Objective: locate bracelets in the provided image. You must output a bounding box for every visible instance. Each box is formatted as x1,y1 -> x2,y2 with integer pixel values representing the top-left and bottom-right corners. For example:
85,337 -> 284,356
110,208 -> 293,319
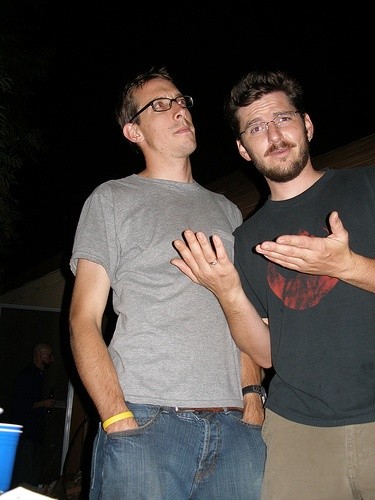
103,411 -> 134,430
242,385 -> 267,403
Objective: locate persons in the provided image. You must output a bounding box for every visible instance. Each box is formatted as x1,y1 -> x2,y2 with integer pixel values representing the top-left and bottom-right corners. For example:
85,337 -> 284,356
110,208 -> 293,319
68,67 -> 268,500
169,72 -> 375,500
12,343 -> 56,479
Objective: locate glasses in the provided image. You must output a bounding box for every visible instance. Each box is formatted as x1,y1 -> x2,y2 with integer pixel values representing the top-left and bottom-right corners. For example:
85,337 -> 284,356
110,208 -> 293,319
237,110 -> 300,137
124,95 -> 193,121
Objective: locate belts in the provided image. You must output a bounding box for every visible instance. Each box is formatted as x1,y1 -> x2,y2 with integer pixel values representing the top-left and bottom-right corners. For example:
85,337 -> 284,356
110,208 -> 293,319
163,401 -> 246,415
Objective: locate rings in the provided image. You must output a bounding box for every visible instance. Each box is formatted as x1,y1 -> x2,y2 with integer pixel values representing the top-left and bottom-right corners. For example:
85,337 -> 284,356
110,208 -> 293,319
209,260 -> 217,265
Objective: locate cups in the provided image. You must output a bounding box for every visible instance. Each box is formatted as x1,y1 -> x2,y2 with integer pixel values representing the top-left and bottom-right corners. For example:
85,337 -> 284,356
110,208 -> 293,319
0,423 -> 23,492
